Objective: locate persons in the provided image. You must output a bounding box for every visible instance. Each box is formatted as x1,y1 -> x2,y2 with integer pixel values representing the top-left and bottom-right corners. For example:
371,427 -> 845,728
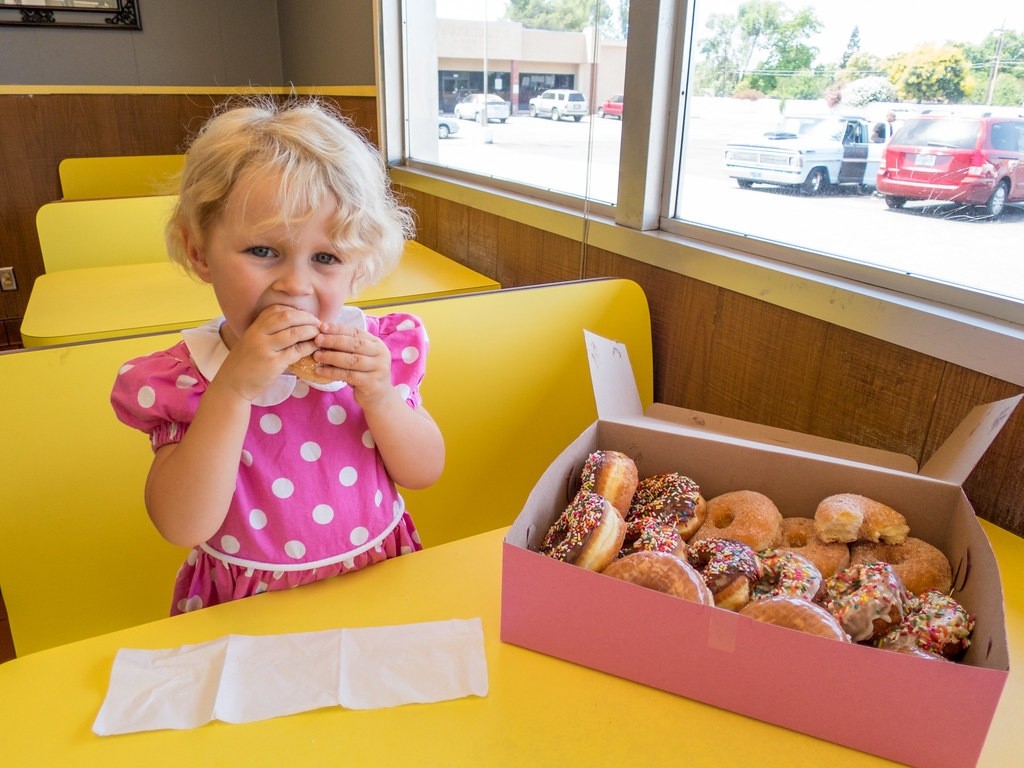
870,112 -> 896,143
109,108 -> 445,617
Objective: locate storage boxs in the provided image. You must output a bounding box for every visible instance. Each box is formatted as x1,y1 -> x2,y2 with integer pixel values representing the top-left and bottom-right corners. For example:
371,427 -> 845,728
500,328 -> 1023,768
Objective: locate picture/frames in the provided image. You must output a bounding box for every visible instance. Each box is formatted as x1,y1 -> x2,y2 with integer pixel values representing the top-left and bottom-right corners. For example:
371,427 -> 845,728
0,0 -> 143,32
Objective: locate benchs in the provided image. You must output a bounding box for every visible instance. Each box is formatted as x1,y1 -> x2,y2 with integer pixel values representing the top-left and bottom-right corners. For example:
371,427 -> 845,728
36,195 -> 183,272
0,277 -> 656,657
59,155 -> 188,198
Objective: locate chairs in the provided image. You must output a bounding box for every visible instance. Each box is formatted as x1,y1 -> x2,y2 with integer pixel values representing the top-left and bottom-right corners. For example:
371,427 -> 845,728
845,125 -> 856,142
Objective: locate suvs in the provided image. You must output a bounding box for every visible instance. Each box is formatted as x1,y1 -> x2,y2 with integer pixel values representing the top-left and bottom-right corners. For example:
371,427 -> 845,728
529,89 -> 588,123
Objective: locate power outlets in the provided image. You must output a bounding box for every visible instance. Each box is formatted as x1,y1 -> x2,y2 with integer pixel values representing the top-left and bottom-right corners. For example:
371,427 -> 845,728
0,267 -> 18,290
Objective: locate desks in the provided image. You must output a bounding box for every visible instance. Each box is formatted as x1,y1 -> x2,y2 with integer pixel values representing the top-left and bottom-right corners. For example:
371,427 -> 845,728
0,516 -> 1023,768
21,239 -> 501,348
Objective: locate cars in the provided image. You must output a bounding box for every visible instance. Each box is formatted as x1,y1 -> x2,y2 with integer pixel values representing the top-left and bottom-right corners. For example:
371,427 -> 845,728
454,93 -> 513,124
439,114 -> 460,139
874,113 -> 1024,221
597,94 -> 624,121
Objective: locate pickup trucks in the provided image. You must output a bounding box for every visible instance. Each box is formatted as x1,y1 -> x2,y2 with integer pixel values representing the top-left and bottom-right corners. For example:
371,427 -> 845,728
724,113 -> 894,197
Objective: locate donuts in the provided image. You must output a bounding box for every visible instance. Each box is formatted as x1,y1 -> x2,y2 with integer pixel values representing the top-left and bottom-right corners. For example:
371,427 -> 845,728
291,348 -> 340,385
542,449 -> 977,662
